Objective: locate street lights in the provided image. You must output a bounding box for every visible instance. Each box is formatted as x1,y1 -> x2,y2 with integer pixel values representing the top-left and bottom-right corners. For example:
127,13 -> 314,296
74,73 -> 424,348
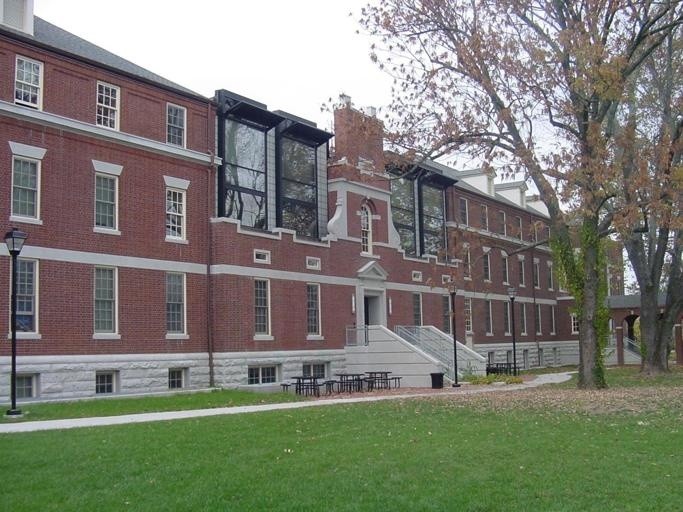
5,226 -> 30,415
507,284 -> 518,376
448,282 -> 461,388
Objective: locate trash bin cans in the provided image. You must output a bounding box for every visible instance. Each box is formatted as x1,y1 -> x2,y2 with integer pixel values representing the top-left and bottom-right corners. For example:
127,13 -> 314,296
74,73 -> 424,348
430,373 -> 444,389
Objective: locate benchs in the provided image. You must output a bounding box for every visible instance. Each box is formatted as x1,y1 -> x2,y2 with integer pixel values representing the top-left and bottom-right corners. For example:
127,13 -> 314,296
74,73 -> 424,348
486,362 -> 522,376
280,372 -> 403,398
